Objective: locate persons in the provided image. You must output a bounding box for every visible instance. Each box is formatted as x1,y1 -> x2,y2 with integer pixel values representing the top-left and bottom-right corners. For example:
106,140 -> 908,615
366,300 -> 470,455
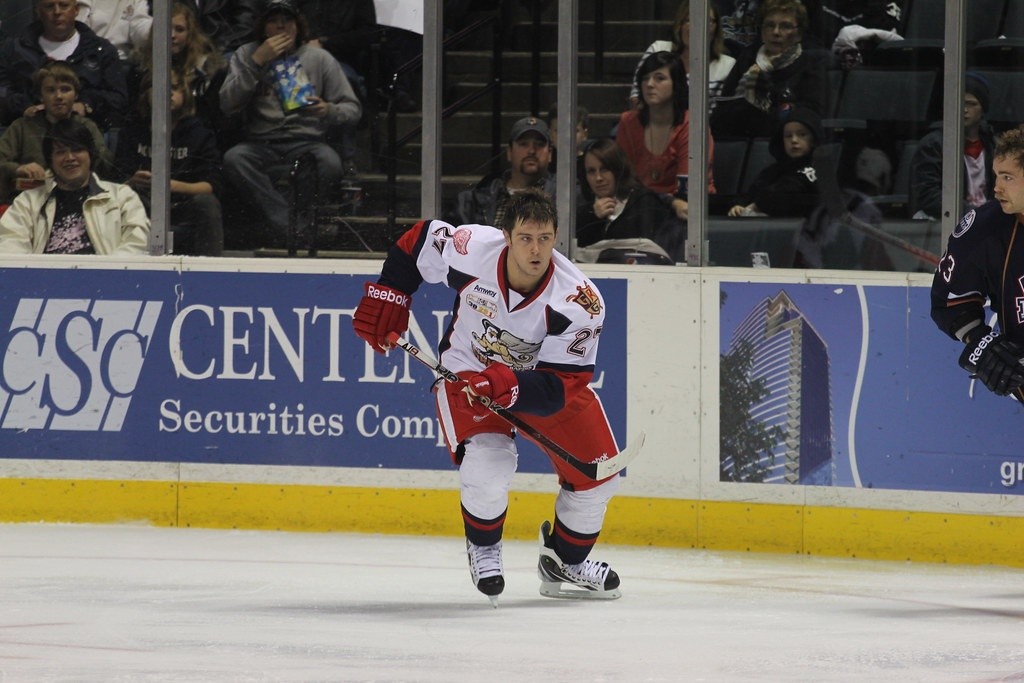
908,75 -> 998,217
294,0 -> 376,176
443,118 -> 687,230
630,0 -> 737,119
114,68 -> 224,257
801,0 -> 901,68
793,145 -> 897,271
727,108 -> 837,218
220,0 -> 363,248
353,189 -> 621,607
577,139 -> 682,265
75,0 -> 154,62
0,62 -> 115,205
614,51 -> 716,199
0,0 -> 128,138
929,124 -> 1024,407
713,0 -> 840,138
0,120 -> 151,255
124,0 -> 226,124
547,102 -> 594,182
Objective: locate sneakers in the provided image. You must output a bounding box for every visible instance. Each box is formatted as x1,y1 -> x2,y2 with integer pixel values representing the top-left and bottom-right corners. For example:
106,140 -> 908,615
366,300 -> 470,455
465,533 -> 505,609
536,521 -> 620,600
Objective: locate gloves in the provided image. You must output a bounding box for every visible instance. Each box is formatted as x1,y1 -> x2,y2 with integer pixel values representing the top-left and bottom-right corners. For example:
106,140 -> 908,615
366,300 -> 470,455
451,363 -> 519,418
351,281 -> 412,354
958,326 -> 1024,395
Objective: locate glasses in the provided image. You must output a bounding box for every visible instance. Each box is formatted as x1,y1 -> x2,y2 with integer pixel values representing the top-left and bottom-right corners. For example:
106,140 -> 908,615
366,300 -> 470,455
761,22 -> 801,33
683,17 -> 717,25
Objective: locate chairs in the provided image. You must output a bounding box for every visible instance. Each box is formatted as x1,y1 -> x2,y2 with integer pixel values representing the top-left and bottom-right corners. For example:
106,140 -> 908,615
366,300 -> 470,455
169,1 -> 1024,251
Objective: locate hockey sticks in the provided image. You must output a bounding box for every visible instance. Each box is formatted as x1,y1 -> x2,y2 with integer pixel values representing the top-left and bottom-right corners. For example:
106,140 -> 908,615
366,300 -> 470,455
386,331 -> 645,481
812,140 -> 942,264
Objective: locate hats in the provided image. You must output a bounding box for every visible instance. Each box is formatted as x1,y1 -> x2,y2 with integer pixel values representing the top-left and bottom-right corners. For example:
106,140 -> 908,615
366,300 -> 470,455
956,78 -> 989,113
511,116 -> 550,141
261,0 -> 298,18
